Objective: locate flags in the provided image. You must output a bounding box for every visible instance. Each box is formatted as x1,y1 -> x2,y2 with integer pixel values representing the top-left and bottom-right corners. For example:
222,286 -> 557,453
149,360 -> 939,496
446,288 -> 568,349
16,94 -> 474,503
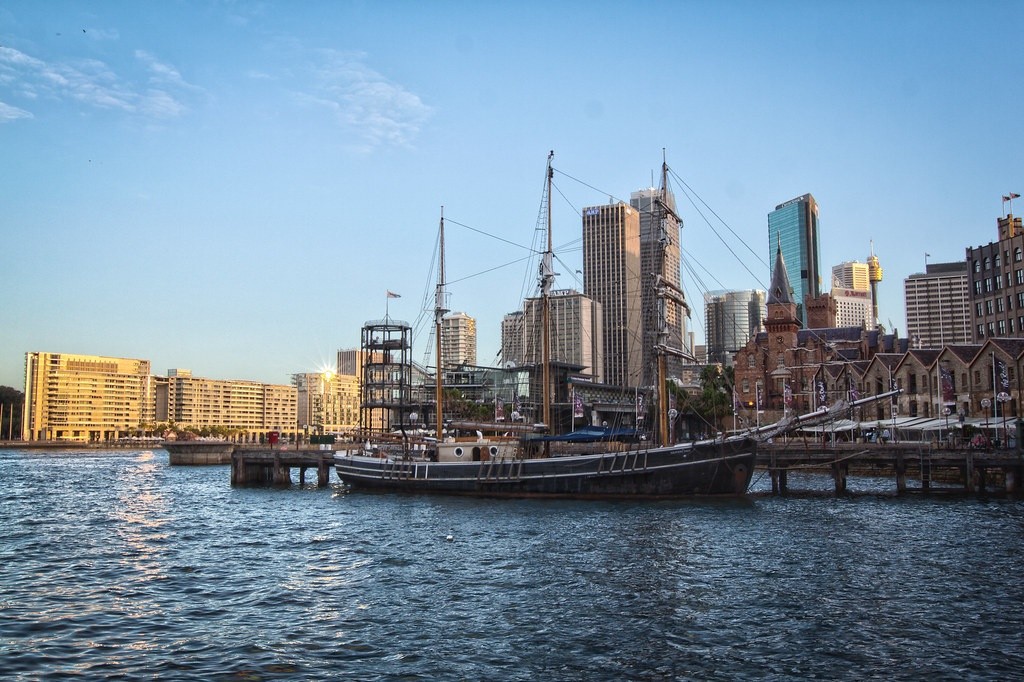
1003,196 -> 1010,201
851,379 -> 856,400
734,394 -> 738,410
576,270 -> 582,274
513,393 -> 522,412
784,385 -> 792,407
926,254 -> 929,256
891,377 -> 897,405
940,365 -> 954,400
1011,193 -> 1020,198
637,395 -> 644,419
574,392 -> 583,418
815,381 -> 826,401
387,291 -> 400,298
497,395 -> 505,420
670,394 -> 676,409
994,357 -> 1008,392
758,386 -> 762,405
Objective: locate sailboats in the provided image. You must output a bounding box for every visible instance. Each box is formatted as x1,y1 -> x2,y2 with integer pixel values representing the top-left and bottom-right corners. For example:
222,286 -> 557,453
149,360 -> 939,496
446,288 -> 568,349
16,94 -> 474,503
330,144 -> 904,510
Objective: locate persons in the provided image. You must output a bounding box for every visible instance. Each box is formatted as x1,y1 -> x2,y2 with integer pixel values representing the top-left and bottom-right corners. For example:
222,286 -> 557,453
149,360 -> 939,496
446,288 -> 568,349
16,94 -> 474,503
1006,425 -> 1010,448
853,425 -> 889,443
